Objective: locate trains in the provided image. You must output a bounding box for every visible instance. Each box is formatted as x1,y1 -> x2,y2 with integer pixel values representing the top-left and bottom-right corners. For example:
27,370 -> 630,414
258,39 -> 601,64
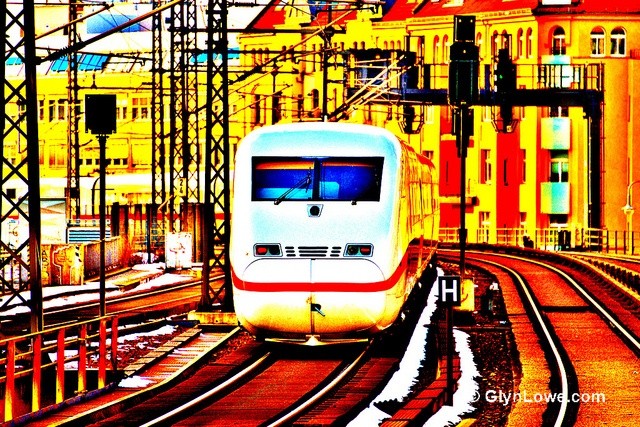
229,123 -> 440,347
